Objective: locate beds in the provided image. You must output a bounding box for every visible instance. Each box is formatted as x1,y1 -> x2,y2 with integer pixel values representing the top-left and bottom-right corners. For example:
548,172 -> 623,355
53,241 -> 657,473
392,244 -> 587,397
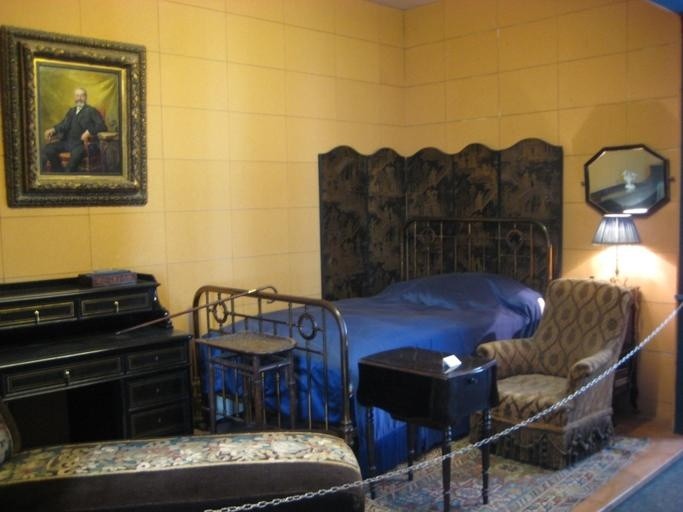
191,214 -> 556,485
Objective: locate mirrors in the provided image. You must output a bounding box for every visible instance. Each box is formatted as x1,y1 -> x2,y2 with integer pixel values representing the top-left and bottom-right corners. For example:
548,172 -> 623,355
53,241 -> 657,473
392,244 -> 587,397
581,142 -> 673,221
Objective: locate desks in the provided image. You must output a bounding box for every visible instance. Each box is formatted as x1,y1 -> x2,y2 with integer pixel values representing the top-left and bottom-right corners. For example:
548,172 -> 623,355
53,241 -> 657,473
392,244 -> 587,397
354,346 -> 501,511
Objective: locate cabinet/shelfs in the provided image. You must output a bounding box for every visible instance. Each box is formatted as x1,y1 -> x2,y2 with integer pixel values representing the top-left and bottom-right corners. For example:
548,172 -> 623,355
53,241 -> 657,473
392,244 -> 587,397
0,265 -> 195,451
197,327 -> 301,439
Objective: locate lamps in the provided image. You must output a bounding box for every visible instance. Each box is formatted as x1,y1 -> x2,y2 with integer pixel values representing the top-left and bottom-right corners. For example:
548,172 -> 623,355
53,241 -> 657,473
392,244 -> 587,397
589,212 -> 644,282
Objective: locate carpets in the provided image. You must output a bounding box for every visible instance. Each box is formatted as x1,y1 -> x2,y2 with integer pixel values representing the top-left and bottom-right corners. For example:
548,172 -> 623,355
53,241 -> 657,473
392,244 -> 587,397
359,408 -> 681,512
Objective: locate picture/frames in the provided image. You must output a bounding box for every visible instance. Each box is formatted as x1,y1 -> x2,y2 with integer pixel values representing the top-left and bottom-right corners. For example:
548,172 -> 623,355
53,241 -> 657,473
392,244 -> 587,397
1,24 -> 151,209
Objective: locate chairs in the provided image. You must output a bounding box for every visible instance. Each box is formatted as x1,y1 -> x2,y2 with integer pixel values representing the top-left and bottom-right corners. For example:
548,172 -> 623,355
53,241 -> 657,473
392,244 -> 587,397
468,276 -> 639,472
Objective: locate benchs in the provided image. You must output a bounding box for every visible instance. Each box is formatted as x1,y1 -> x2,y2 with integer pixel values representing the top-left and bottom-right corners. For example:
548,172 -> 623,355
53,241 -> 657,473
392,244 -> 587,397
2,407 -> 372,511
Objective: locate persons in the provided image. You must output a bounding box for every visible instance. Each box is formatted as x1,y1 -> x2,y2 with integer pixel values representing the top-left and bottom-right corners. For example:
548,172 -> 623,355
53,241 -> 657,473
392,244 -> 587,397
43,88 -> 107,174
